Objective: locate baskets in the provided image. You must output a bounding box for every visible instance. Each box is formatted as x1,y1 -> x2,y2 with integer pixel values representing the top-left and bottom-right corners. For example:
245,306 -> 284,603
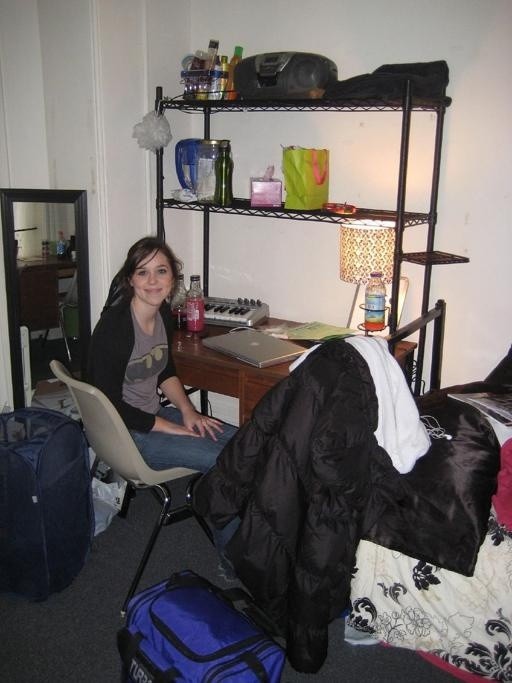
181,69 -> 240,100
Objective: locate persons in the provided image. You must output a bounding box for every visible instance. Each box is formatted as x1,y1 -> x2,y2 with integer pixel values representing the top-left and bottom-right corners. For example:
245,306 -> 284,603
87,235 -> 241,481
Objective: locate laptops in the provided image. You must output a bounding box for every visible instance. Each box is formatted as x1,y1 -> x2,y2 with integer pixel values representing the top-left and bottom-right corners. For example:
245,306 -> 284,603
201,329 -> 308,369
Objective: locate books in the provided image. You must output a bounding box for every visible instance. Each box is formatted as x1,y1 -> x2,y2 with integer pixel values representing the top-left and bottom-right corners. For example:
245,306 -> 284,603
464,393 -> 511,423
446,385 -> 512,428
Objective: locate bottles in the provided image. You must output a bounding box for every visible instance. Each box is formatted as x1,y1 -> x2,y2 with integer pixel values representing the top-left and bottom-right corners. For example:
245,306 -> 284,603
213,138 -> 235,206
55,231 -> 67,256
171,273 -> 188,329
364,271 -> 386,331
183,38 -> 245,100
185,274 -> 205,331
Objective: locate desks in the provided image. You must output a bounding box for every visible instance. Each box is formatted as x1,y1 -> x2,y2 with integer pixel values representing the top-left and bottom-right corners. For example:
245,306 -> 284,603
168,318 -> 416,429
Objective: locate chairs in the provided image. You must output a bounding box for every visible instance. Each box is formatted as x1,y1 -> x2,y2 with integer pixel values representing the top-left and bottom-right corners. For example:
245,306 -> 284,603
56,294 -> 77,363
47,361 -> 227,613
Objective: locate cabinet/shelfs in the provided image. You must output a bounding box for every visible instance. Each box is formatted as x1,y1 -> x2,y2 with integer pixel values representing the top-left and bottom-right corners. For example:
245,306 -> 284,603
154,83 -> 470,361
17,255 -> 72,329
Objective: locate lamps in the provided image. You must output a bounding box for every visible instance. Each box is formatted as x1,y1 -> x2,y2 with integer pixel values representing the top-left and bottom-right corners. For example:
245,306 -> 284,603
338,220 -> 397,285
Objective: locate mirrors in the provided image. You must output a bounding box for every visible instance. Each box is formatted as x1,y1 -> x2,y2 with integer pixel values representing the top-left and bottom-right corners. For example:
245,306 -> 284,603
1,187 -> 92,431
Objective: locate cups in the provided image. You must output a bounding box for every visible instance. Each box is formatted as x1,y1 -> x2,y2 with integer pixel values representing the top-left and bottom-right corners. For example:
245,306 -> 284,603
175,138 -> 222,202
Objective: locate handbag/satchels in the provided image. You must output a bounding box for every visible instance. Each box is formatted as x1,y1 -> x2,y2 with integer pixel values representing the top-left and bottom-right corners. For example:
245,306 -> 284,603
0,407 -> 95,604
282,146 -> 331,211
116,568 -> 285,682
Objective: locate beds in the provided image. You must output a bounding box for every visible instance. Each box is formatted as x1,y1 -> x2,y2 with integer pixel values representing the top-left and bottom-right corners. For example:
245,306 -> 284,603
310,295 -> 509,683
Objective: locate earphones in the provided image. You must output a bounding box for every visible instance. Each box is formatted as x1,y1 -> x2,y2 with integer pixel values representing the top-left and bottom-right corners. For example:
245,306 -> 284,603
443,433 -> 452,440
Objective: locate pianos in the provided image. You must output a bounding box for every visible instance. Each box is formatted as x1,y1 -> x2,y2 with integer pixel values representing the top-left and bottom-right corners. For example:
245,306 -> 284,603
204,296 -> 269,329
